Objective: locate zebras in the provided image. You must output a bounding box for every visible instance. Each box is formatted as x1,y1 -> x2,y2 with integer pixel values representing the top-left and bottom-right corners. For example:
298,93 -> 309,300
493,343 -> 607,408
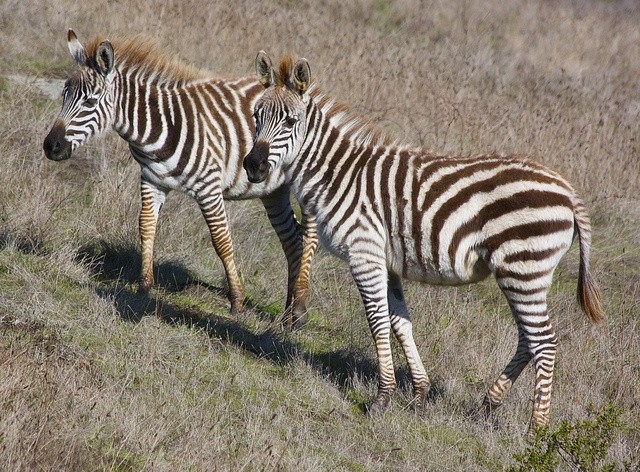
242,47 -> 609,456
41,27 -> 318,335
